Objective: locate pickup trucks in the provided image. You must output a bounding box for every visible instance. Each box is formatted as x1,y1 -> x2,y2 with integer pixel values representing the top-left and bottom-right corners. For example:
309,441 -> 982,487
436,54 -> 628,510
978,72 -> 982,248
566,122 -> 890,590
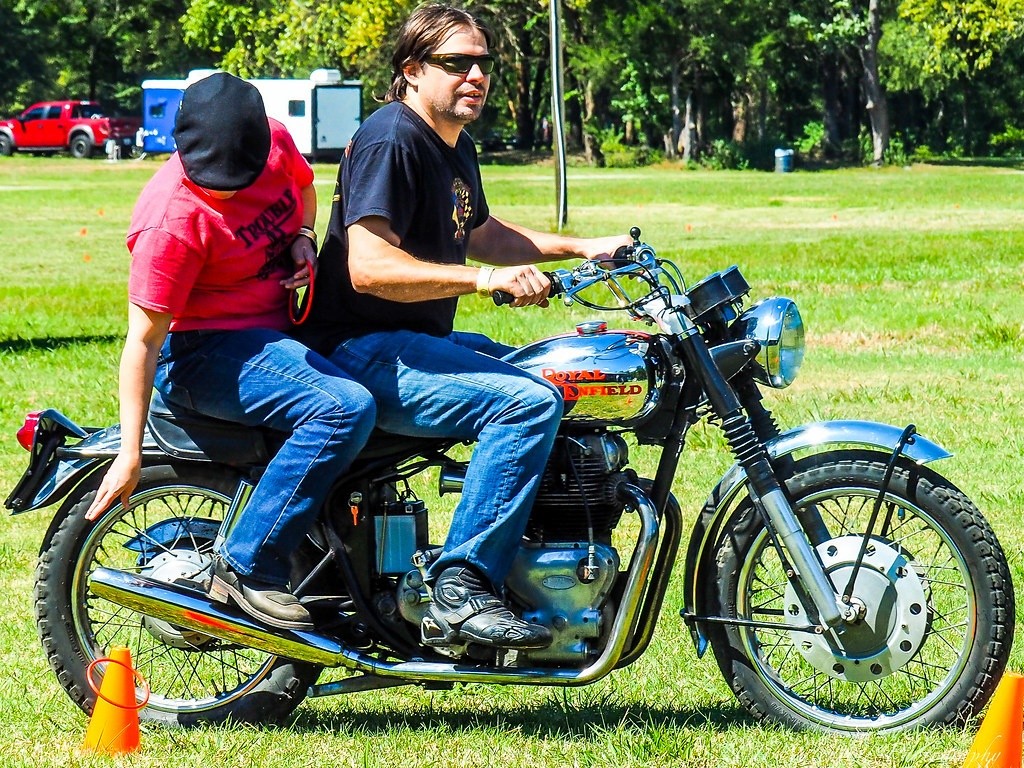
0,100 -> 141,158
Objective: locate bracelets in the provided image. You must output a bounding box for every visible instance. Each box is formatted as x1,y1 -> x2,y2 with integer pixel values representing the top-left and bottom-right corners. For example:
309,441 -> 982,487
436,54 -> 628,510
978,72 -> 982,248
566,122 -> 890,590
296,224 -> 318,257
477,266 -> 494,299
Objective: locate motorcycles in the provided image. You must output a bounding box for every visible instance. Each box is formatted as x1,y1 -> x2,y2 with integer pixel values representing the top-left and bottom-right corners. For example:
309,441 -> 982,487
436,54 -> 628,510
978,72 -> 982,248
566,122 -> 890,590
0,222 -> 1016,739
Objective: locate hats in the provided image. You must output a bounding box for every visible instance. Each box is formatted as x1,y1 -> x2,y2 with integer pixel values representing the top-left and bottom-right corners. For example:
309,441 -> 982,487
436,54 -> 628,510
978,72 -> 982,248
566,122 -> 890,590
173,72 -> 271,191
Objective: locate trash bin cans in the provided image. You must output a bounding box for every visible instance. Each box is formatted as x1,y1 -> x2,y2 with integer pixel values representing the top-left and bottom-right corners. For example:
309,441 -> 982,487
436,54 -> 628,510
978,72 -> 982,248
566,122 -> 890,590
773,148 -> 796,173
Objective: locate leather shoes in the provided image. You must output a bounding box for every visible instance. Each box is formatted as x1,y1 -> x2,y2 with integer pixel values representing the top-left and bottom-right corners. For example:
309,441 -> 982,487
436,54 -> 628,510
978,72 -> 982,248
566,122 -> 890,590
209,556 -> 314,630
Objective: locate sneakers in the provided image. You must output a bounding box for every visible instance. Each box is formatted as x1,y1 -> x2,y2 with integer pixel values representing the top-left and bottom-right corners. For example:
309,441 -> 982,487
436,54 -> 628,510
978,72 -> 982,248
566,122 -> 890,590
420,567 -> 552,649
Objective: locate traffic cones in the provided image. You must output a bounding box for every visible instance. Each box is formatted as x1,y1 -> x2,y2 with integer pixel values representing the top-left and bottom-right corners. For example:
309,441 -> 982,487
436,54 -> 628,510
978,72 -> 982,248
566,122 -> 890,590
959,674 -> 1024,768
80,645 -> 141,754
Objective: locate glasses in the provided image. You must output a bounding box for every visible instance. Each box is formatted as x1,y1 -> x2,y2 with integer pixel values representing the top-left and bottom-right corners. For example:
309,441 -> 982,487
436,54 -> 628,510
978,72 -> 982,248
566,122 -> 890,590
421,53 -> 496,75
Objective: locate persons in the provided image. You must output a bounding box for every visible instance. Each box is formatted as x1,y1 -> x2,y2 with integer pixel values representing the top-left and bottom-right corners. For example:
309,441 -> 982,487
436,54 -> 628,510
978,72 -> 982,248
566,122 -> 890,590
83,73 -> 378,632
283,6 -> 639,648
